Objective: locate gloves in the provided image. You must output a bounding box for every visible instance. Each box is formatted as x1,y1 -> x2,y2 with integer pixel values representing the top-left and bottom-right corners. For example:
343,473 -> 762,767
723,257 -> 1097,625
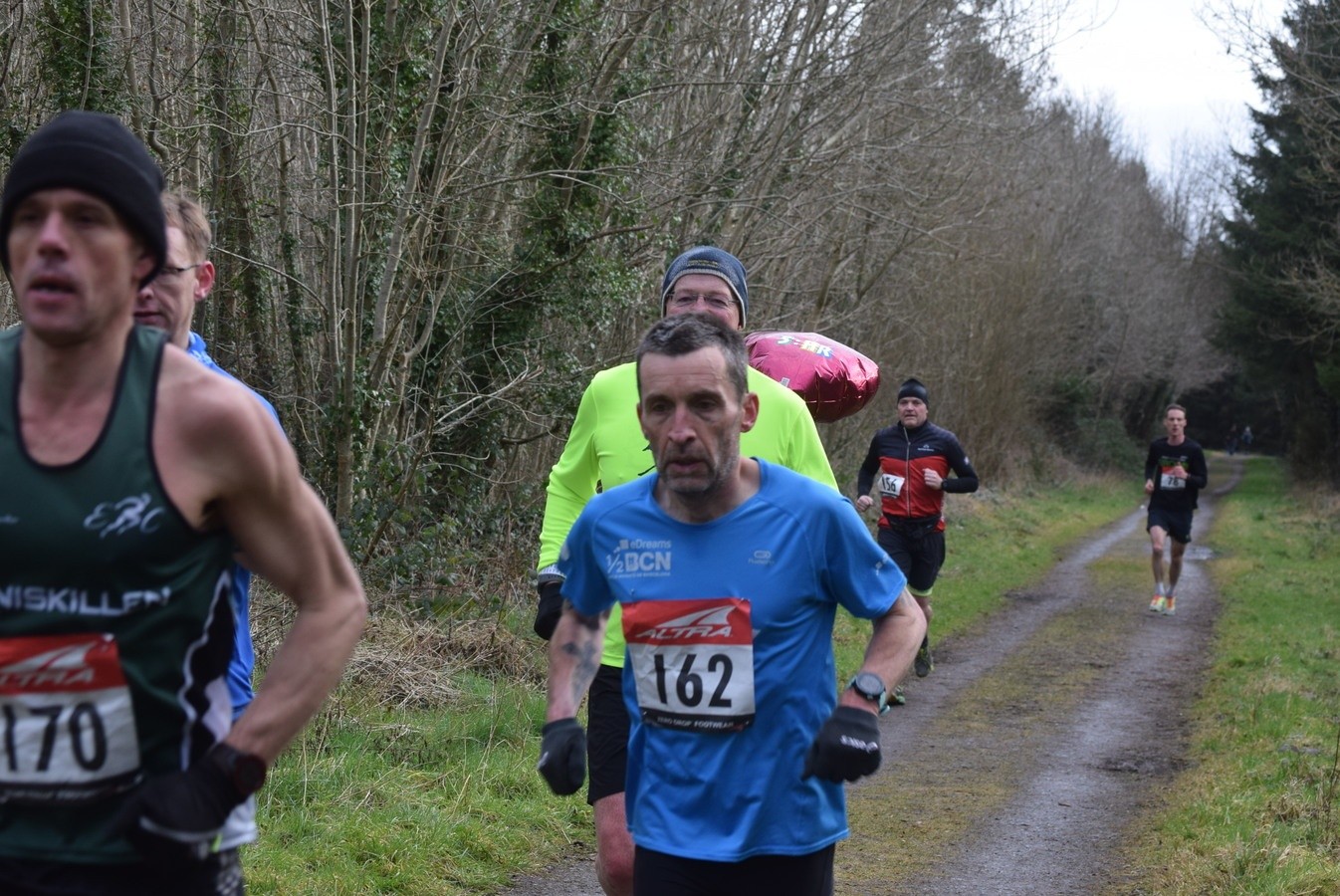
533,583 -> 563,641
801,706 -> 881,784
97,738 -> 267,882
536,717 -> 588,797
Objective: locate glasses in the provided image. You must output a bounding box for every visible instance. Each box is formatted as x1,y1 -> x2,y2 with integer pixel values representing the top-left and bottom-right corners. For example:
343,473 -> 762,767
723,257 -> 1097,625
152,264 -> 199,286
667,292 -> 739,312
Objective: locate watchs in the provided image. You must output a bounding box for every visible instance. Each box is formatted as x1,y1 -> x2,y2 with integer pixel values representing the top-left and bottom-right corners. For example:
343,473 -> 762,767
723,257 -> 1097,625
1186,473 -> 1191,480
941,478 -> 948,490
847,671 -> 890,717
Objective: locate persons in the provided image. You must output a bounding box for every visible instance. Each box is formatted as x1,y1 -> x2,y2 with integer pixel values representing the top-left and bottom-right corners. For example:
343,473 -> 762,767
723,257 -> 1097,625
129,193 -> 260,896
856,377 -> 979,705
1144,404 -> 1208,616
540,312 -> 929,896
0,111 -> 366,896
534,247 -> 842,896
1226,425 -> 1253,456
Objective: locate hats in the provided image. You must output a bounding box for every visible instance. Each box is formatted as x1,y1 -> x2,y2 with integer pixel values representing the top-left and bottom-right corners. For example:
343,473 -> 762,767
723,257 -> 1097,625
661,247 -> 748,331
0,111 -> 167,291
898,378 -> 928,407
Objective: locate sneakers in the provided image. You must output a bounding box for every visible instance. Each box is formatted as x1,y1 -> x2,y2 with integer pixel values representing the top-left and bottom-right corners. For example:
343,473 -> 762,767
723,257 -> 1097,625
1163,595 -> 1176,615
1149,594 -> 1165,611
914,648 -> 933,678
887,685 -> 906,705
